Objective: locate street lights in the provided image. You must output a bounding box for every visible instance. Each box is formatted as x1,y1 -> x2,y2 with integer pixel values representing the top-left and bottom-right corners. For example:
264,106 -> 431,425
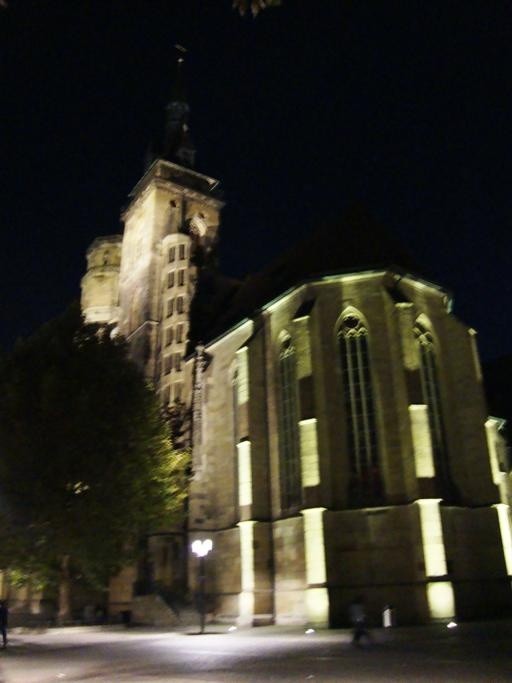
188,536 -> 215,634
